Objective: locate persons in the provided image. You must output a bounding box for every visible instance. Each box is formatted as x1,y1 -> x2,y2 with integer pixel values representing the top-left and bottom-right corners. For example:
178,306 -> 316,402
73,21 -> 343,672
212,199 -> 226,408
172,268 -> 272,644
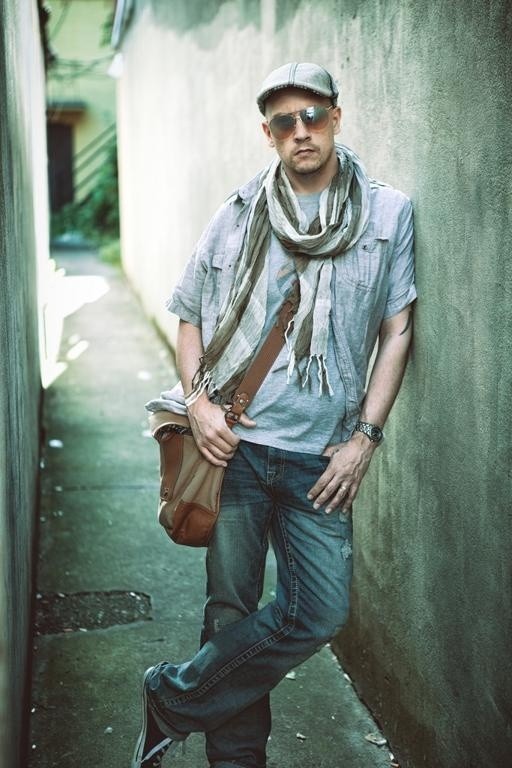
132,62 -> 416,768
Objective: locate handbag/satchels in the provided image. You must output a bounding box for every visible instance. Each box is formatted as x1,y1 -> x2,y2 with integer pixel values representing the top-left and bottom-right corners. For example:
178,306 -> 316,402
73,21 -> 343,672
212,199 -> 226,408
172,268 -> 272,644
150,411 -> 226,547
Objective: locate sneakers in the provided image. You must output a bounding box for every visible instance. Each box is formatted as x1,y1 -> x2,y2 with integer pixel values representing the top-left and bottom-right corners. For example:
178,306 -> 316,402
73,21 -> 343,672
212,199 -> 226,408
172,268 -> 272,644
132,667 -> 172,768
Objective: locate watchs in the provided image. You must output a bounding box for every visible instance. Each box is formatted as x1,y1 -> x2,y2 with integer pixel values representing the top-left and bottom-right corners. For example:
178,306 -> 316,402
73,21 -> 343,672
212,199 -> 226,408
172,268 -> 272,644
353,421 -> 383,442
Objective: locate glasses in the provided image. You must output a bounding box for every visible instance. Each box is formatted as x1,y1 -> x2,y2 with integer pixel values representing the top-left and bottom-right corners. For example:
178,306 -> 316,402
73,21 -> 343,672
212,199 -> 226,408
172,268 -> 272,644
267,104 -> 333,140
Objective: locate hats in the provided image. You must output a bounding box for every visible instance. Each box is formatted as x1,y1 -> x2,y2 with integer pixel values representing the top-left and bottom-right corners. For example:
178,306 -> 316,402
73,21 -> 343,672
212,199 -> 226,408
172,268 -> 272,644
256,62 -> 339,113
